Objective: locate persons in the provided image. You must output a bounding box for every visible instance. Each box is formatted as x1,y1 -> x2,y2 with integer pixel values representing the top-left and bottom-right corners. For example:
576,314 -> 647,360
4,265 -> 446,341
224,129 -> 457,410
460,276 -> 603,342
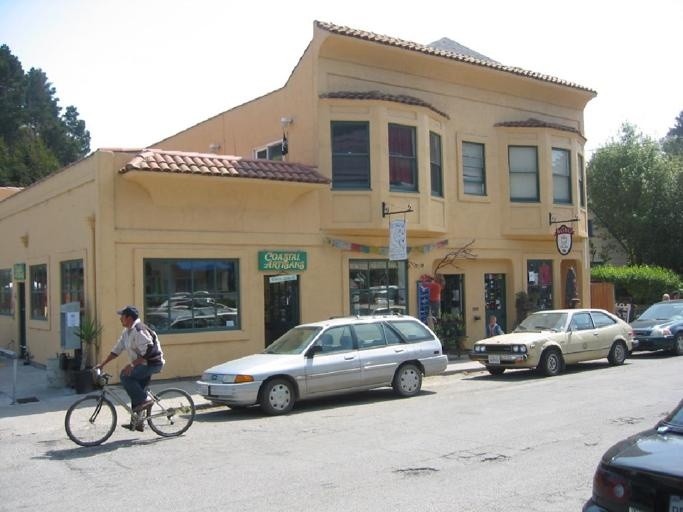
485,315 -> 504,337
92,305 -> 166,432
671,290 -> 680,299
419,273 -> 443,317
662,293 -> 670,301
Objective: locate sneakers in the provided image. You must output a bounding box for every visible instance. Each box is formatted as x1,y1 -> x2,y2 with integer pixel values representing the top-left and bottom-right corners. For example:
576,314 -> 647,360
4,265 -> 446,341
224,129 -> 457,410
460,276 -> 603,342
121,424 -> 145,432
133,399 -> 154,413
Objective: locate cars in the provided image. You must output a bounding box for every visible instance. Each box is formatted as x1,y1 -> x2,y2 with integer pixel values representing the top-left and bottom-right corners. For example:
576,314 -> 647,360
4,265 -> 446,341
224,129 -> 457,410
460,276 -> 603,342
146,292 -> 238,329
583,398 -> 682,512
628,299 -> 682,356
469,309 -> 634,377
194,311 -> 449,416
350,285 -> 406,315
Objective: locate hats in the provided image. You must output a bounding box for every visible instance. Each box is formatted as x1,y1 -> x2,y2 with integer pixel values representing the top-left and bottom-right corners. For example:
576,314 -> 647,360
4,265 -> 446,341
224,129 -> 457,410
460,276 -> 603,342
116,306 -> 138,318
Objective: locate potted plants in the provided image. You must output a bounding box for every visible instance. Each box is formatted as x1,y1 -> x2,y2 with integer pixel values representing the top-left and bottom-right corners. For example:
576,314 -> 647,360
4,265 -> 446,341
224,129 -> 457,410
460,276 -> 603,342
73,314 -> 105,369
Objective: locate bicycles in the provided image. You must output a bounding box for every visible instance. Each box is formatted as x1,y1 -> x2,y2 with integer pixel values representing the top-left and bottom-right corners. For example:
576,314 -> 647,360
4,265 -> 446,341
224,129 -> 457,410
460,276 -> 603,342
66,367 -> 195,447
